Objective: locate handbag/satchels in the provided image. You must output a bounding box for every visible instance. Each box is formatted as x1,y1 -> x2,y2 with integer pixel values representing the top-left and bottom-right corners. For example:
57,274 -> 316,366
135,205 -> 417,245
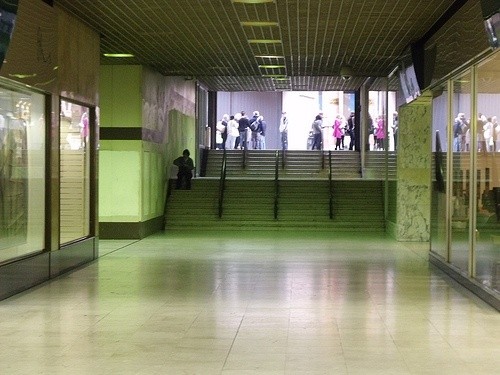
216,123 -> 225,133
231,127 -> 240,137
250,121 -> 259,131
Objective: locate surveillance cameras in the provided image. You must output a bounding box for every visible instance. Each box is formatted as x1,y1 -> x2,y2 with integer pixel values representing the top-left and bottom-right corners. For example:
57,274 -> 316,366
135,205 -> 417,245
340,70 -> 352,80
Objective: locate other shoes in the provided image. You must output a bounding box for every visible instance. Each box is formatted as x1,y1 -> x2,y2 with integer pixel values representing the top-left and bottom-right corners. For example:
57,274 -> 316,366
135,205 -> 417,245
186,183 -> 190,189
175,187 -> 180,189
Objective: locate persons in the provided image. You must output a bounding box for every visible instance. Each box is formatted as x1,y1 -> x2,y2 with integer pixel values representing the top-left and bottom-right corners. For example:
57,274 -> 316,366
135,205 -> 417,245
311,111 -> 398,151
81,114 -> 88,148
452,113 -> 498,152
216,110 -> 268,149
173,148 -> 194,190
279,111 -> 289,150
